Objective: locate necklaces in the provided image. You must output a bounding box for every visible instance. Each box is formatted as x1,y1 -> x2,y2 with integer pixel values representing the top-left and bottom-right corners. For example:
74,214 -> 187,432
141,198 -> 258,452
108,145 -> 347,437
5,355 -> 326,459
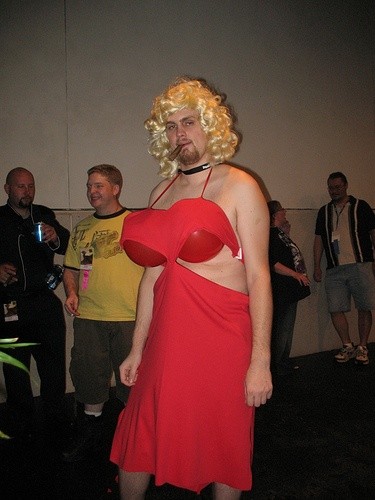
176,163 -> 210,175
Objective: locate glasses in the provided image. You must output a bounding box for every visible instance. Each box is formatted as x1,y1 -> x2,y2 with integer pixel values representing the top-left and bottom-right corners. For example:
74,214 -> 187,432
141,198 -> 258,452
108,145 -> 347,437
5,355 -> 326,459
328,183 -> 346,190
275,208 -> 287,213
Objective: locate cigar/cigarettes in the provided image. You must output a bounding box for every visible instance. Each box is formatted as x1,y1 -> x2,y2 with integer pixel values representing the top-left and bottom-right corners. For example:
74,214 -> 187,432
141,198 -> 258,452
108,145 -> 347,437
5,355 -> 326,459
169,144 -> 183,160
4,275 -> 13,286
71,309 -> 75,316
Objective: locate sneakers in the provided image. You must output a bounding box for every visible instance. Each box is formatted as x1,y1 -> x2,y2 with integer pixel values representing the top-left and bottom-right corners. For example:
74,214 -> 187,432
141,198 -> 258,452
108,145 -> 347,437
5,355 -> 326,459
355,346 -> 369,365
334,342 -> 357,363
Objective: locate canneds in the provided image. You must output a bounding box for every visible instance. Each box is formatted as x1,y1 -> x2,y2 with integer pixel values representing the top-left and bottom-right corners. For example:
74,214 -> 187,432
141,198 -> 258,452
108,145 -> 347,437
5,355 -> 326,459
33,221 -> 46,243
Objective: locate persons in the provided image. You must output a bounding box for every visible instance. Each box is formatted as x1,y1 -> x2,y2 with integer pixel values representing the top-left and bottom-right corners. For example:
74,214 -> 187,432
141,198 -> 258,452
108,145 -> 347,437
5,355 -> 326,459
0,168 -> 70,457
268,200 -> 314,372
109,79 -> 273,500
63,164 -> 145,435
312,172 -> 375,364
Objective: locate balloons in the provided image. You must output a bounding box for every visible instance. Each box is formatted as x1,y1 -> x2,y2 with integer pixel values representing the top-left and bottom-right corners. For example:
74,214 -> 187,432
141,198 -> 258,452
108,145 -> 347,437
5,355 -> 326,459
122,239 -> 166,268
178,231 -> 225,265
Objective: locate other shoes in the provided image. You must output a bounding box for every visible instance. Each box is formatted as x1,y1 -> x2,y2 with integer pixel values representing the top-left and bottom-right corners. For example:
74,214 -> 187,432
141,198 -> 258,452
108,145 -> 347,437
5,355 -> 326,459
270,362 -> 297,377
62,428 -> 102,453
